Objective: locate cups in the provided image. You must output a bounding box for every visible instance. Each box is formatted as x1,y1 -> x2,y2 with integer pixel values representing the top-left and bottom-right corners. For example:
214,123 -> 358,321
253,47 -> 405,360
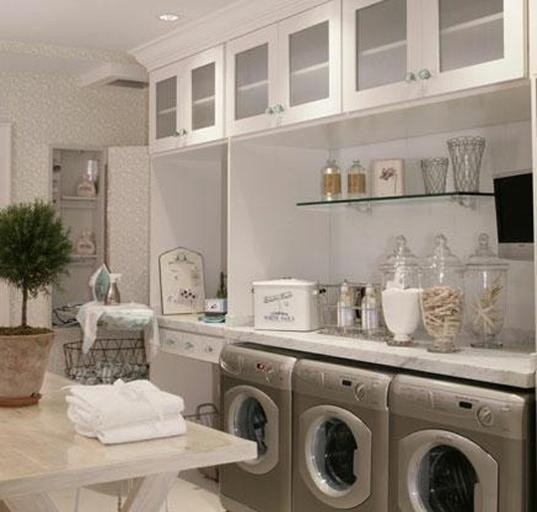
448,135 -> 486,195
420,157 -> 448,193
83,159 -> 98,186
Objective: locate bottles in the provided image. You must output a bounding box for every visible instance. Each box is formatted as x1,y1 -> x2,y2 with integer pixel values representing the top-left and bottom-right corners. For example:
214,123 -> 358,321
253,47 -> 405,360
320,159 -> 364,197
360,284 -> 379,332
381,234 -> 507,351
76,231 -> 94,255
336,280 -> 355,324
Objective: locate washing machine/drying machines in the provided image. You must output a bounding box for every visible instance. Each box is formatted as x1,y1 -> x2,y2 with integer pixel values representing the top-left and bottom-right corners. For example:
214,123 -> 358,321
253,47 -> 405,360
390,369 -> 532,512
292,356 -> 392,512
217,341 -> 297,512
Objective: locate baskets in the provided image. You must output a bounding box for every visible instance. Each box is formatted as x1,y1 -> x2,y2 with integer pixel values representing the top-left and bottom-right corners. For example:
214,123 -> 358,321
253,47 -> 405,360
63,337 -> 147,385
316,281 -> 381,339
184,404 -> 222,481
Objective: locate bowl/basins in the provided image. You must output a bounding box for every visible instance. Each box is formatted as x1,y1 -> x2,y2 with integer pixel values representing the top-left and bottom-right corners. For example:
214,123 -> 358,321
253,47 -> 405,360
203,297 -> 225,316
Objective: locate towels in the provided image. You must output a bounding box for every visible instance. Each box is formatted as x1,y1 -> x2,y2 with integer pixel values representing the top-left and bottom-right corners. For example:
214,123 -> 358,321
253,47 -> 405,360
64,379 -> 189,444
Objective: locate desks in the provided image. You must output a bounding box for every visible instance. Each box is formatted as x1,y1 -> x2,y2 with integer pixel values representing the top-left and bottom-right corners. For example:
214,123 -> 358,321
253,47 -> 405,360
0,390 -> 258,512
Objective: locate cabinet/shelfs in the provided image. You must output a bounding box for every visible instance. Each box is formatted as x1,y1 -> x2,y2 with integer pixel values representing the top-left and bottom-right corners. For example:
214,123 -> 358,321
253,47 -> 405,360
148,43 -> 223,161
156,326 -> 222,363
223,2 -> 341,143
51,146 -> 106,331
340,0 -> 528,122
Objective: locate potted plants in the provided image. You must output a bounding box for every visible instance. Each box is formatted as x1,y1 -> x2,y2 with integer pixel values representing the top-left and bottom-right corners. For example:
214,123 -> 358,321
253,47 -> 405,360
0,198 -> 71,408
